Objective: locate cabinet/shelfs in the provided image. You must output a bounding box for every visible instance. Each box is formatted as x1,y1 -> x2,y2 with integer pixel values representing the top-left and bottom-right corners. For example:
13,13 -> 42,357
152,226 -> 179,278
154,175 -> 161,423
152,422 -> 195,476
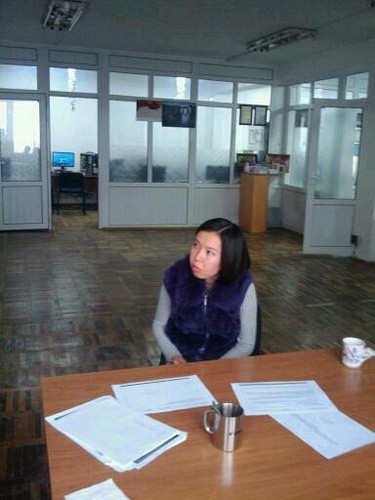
239,171 -> 283,234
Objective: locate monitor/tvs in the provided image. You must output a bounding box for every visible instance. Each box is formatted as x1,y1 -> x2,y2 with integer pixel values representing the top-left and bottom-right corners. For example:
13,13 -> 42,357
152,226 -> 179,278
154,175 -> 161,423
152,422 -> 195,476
50,150 -> 78,171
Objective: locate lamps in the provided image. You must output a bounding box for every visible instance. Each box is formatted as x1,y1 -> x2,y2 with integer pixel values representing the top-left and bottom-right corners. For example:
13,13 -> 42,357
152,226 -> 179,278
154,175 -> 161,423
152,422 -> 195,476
246,27 -> 317,54
41,0 -> 88,32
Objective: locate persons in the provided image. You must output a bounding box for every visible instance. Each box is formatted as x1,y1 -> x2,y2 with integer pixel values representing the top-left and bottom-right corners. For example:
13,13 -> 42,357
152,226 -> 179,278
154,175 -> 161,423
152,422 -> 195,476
153,218 -> 257,366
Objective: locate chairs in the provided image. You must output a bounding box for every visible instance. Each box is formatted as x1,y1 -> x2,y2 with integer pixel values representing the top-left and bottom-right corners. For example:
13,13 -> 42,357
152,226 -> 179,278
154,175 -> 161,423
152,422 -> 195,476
57,173 -> 88,216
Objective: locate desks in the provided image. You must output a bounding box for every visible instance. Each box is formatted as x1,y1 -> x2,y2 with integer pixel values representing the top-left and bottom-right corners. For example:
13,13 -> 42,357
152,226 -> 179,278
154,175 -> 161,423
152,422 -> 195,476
50,175 -> 98,208
39,340 -> 375,500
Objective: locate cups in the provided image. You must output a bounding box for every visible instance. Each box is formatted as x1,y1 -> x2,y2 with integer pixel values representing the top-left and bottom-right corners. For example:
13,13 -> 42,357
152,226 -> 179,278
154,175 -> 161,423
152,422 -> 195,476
203,401 -> 244,453
341,336 -> 364,368
